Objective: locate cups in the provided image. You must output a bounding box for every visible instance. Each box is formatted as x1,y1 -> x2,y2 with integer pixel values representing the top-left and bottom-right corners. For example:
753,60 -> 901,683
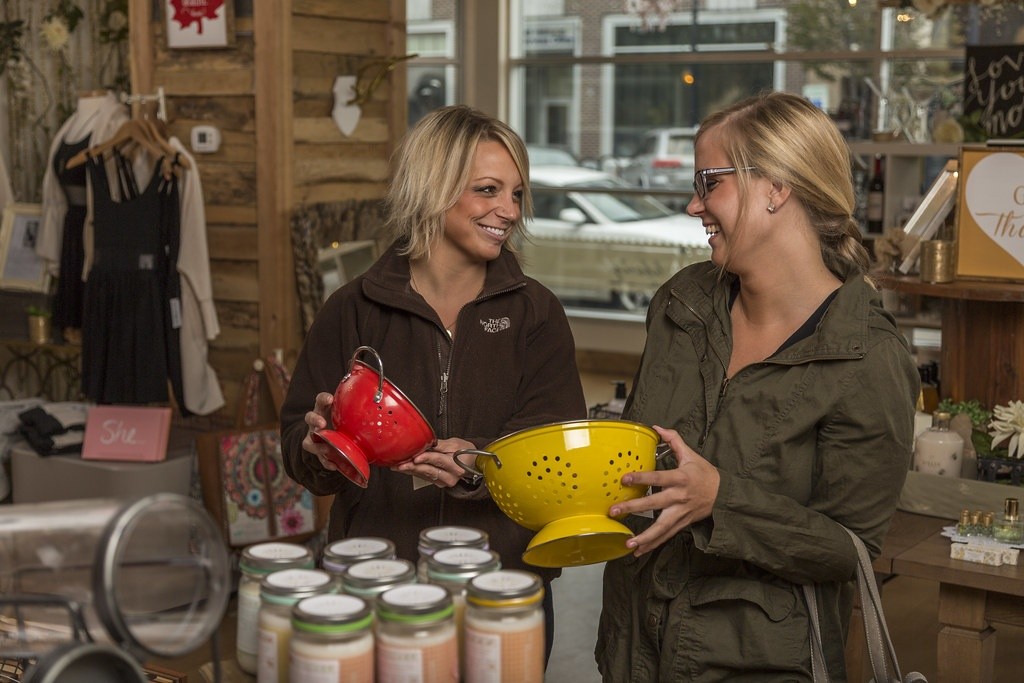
920,241 -> 954,283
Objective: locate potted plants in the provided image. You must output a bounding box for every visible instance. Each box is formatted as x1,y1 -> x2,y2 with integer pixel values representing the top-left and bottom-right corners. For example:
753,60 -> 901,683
25,303 -> 55,343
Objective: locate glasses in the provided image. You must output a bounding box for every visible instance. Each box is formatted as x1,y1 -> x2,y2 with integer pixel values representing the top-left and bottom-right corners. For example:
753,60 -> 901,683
692,166 -> 762,199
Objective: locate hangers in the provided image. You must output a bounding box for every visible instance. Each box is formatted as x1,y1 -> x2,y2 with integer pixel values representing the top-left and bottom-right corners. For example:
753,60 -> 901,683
66,91 -> 192,180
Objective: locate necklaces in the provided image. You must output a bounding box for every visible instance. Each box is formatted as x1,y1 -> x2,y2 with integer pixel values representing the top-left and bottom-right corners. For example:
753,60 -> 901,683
409,263 -> 483,338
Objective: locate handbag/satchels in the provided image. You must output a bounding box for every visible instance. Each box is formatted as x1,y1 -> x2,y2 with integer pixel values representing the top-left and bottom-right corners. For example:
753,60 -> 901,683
194,364 -> 329,551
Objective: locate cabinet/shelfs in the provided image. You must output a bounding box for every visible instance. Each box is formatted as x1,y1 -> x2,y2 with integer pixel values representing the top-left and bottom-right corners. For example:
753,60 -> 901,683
846,136 -> 957,344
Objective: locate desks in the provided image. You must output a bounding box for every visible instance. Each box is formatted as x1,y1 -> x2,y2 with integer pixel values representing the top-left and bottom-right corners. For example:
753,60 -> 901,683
0,336 -> 82,402
845,510 -> 1024,683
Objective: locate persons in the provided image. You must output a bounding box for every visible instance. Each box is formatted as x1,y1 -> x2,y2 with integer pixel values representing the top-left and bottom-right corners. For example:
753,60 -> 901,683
595,92 -> 921,683
280,105 -> 588,674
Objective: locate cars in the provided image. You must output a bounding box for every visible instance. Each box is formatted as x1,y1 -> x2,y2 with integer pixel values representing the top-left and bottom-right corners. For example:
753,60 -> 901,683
506,165 -> 712,302
621,127 -> 698,204
525,145 -> 579,166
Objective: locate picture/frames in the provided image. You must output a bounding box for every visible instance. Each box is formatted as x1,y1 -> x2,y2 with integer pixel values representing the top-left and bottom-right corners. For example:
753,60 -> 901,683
0,200 -> 52,294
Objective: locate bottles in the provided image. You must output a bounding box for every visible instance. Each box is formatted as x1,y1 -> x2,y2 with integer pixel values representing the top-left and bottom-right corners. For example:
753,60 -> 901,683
913,409 -> 965,478
955,498 -> 1024,544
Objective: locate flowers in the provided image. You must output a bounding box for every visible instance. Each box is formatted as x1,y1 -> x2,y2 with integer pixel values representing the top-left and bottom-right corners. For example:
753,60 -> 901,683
987,400 -> 1024,458
